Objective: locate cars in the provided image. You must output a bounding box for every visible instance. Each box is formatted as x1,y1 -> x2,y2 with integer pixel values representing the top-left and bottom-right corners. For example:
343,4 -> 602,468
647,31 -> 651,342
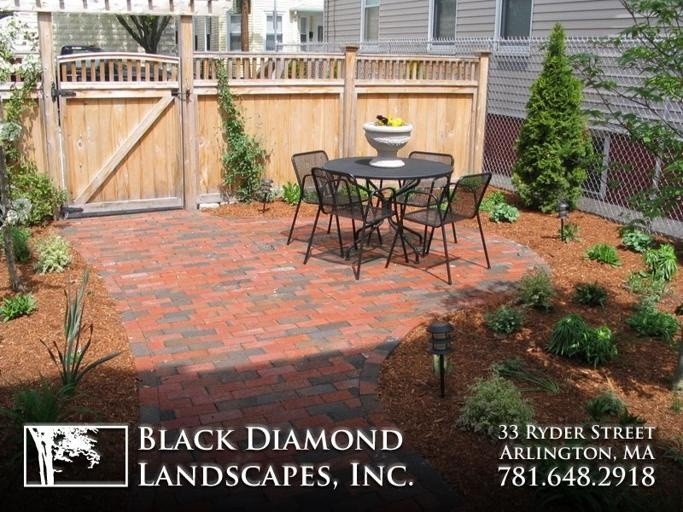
57,42 -> 171,81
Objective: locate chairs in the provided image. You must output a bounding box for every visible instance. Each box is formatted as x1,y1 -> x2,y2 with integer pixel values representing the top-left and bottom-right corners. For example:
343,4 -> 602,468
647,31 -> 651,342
303,166 -> 409,280
386,151 -> 457,258
287,150 -> 382,251
385,171 -> 492,285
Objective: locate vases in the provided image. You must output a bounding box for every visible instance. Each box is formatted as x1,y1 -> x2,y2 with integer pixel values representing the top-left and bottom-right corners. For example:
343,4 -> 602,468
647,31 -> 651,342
362,120 -> 413,168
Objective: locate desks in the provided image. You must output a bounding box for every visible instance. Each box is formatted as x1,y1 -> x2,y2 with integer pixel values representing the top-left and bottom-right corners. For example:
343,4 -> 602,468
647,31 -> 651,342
322,156 -> 454,264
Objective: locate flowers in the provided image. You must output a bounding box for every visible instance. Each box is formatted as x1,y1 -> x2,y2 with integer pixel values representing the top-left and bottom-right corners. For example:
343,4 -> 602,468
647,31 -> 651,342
375,114 -> 405,128
